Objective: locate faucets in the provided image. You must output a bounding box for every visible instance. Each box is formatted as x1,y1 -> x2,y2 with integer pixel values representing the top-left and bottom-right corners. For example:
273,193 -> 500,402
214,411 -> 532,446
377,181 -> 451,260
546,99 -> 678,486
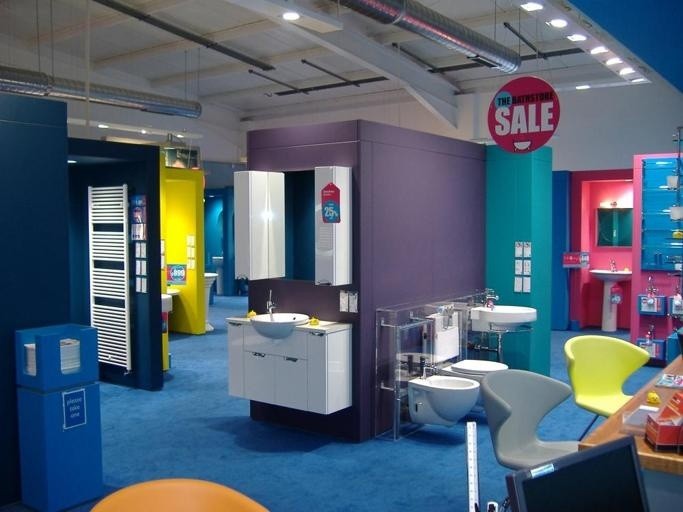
648,324 -> 655,335
267,290 -> 281,313
610,258 -> 616,272
420,364 -> 437,379
484,288 -> 499,308
647,275 -> 656,293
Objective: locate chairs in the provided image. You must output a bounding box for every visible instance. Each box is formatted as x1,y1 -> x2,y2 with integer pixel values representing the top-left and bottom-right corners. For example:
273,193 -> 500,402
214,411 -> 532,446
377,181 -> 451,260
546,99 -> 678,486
482,331 -> 654,467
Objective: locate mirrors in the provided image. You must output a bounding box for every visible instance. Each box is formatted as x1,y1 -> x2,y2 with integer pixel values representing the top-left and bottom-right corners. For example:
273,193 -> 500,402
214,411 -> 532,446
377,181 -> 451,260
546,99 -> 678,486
594,206 -> 633,249
267,167 -> 314,281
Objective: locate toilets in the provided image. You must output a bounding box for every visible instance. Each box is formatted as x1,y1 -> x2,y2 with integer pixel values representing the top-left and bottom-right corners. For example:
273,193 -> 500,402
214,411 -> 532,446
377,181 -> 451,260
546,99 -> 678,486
425,311 -> 509,407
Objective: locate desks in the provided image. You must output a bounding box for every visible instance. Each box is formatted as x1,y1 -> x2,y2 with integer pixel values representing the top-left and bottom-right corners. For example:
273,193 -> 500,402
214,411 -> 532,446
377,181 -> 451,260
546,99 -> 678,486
578,350 -> 681,509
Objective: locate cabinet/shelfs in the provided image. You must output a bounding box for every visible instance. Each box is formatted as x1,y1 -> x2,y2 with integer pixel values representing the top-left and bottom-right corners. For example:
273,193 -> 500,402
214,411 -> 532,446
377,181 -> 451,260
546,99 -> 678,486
232,169 -> 267,282
226,320 -> 244,399
305,330 -> 353,415
313,164 -> 355,286
12,322 -> 104,511
242,351 -> 307,411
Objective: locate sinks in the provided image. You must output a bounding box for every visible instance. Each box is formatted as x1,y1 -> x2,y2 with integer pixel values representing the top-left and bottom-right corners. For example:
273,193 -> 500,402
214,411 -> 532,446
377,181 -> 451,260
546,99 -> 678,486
212,257 -> 223,265
250,313 -> 309,338
590,269 -> 632,281
408,373 -> 481,427
471,304 -> 537,327
